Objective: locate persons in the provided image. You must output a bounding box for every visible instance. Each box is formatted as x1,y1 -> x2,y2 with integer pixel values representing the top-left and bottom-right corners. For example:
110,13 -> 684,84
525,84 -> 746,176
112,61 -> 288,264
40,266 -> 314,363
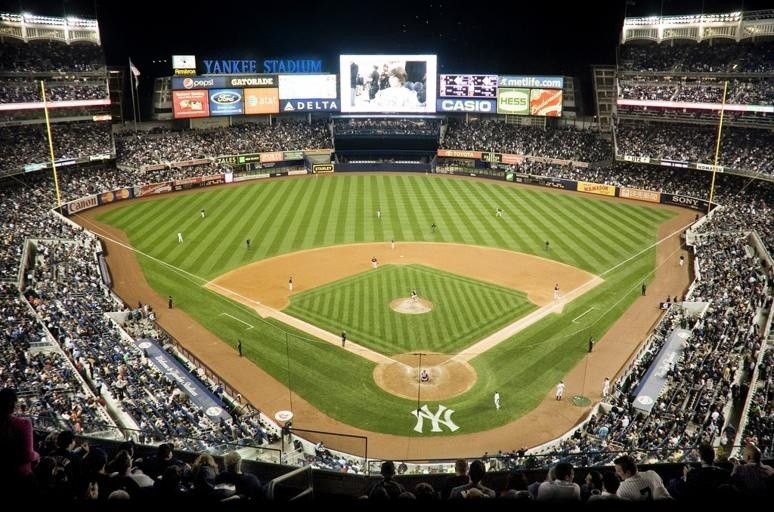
0,43 -> 774,512
